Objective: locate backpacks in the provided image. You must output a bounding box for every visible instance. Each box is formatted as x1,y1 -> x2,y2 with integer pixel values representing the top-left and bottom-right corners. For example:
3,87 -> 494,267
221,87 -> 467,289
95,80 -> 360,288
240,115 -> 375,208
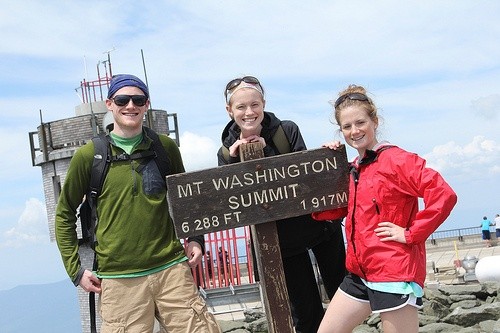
77,124 -> 175,249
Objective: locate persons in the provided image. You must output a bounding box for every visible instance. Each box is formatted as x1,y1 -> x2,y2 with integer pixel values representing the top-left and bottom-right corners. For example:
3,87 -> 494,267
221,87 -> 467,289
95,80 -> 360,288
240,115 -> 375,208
480,216 -> 493,247
54,74 -> 230,333
312,84 -> 457,333
493,213 -> 500,242
216,75 -> 347,333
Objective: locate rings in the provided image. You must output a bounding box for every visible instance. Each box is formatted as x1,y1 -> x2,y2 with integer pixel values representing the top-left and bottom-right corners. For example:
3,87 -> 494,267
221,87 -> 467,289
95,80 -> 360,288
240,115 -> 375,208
388,231 -> 392,237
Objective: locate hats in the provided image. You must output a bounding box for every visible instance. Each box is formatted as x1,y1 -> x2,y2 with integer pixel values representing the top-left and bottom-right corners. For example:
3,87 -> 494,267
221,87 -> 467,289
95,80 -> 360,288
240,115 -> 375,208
224,77 -> 264,104
107,73 -> 149,99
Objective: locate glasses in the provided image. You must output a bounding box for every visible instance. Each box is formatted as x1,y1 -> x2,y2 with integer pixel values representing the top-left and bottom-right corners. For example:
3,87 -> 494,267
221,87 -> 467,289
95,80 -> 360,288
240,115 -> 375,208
110,94 -> 148,107
225,76 -> 265,101
334,92 -> 372,108
